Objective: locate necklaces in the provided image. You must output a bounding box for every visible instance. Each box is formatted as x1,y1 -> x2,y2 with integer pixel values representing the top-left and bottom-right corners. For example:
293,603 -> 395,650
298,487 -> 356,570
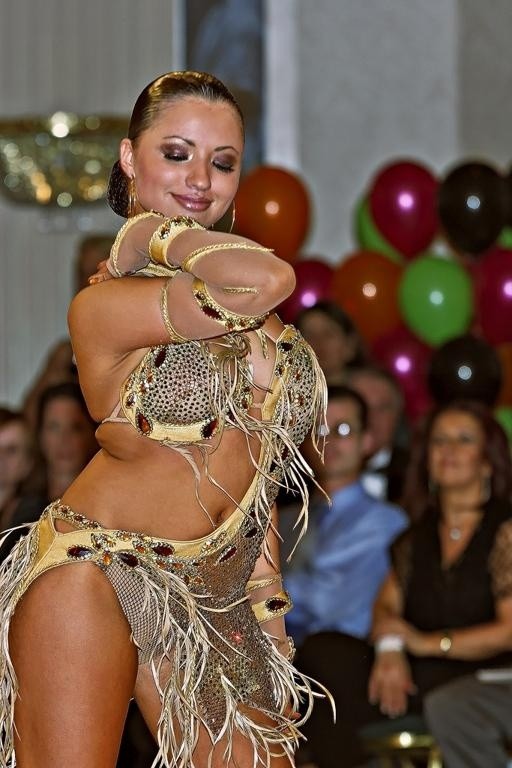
448,528 -> 462,540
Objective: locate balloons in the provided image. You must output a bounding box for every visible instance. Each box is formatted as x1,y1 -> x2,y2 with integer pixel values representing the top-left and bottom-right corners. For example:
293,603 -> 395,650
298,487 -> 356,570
281,257 -> 332,324
235,165 -> 311,261
340,252 -> 402,338
398,258 -> 472,348
467,246 -> 512,438
358,162 -> 512,259
431,338 -> 501,414
372,331 -> 434,422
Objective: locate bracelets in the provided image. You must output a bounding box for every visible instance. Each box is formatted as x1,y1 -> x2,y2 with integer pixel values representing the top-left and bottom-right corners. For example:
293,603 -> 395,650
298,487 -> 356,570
374,637 -> 405,653
441,629 -> 451,659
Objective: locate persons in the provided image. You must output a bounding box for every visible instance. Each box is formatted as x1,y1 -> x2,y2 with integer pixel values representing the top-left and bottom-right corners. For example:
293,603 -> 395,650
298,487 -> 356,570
303,399 -> 511,768
0,341 -> 100,560
276,386 -> 412,657
299,307 -> 413,501
0,70 -> 332,766
